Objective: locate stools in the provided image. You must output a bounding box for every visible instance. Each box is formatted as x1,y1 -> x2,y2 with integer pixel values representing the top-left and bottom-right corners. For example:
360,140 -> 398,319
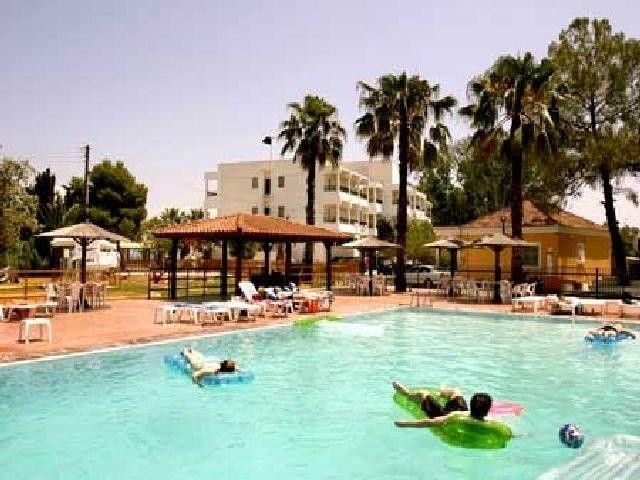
18,318 -> 52,344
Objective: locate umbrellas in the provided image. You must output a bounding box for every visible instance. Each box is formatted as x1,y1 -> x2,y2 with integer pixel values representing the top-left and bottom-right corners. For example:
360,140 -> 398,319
339,234 -> 403,297
422,236 -> 478,297
461,229 -> 541,304
31,221 -> 132,310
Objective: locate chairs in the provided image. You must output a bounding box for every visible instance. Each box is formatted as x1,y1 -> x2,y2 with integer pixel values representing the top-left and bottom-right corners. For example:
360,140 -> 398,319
157,281 -> 333,326
346,274 -> 640,318
0,280 -> 107,322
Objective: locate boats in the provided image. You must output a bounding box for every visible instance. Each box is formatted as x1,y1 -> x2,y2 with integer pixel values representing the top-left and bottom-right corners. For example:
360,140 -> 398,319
393,386 -> 511,450
532,432 -> 640,480
163,350 -> 254,387
292,313 -> 342,327
309,319 -> 386,341
585,329 -> 628,343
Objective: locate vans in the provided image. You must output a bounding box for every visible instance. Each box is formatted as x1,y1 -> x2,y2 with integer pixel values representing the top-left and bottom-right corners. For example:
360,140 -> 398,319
372,256 -> 393,276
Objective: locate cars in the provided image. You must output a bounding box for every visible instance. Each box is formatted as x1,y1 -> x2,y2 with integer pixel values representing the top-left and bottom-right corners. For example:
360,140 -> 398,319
384,264 -> 450,287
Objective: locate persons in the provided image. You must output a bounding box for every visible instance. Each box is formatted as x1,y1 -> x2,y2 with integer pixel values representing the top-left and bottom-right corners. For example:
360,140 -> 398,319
584,320 -> 638,348
181,345 -> 242,389
391,378 -> 537,440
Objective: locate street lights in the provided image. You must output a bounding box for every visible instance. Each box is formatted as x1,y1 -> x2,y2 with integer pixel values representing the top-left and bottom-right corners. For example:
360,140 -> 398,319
19,225 -> 33,302
